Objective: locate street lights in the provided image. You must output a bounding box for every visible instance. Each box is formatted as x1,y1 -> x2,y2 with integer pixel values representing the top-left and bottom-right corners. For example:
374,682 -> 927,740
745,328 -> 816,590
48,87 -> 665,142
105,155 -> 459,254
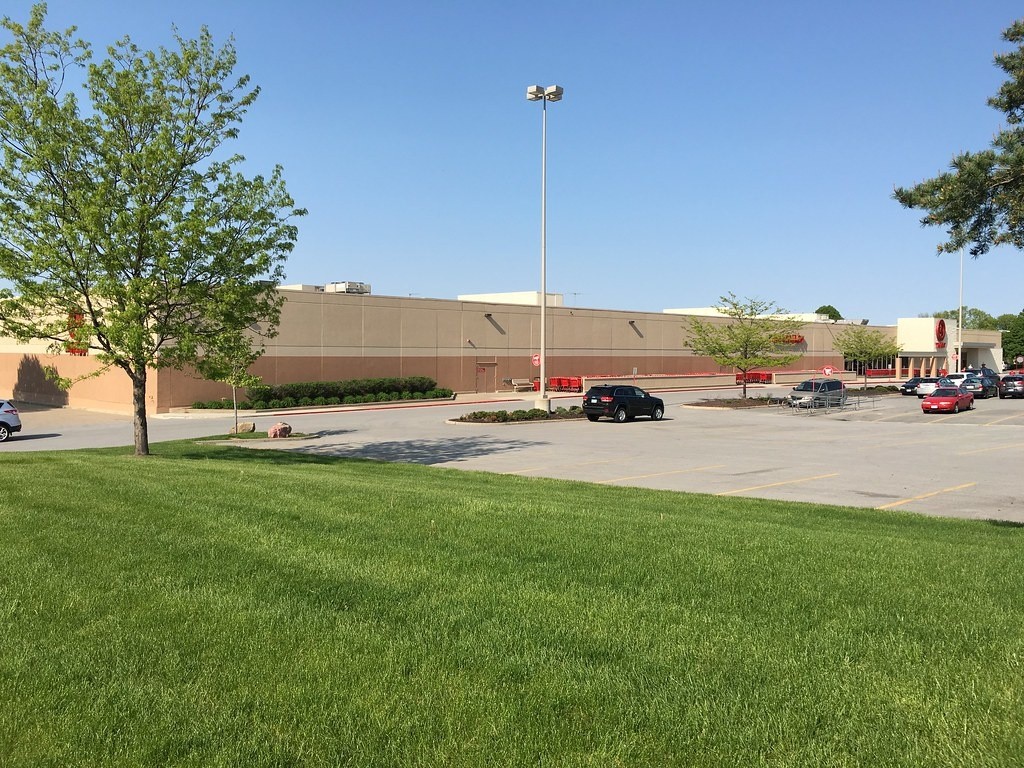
528,83 -> 567,412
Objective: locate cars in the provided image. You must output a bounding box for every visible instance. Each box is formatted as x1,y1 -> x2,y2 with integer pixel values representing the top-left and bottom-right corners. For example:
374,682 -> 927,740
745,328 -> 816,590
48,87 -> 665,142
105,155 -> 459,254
917,377 -> 957,399
921,387 -> 975,415
959,377 -> 998,400
998,374 -> 1024,399
900,377 -> 922,396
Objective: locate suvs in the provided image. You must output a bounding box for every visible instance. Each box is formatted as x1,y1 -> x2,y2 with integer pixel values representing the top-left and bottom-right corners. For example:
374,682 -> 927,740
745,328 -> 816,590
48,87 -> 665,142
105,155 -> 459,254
945,372 -> 978,388
783,378 -> 848,410
965,368 -> 1001,381
582,384 -> 665,423
